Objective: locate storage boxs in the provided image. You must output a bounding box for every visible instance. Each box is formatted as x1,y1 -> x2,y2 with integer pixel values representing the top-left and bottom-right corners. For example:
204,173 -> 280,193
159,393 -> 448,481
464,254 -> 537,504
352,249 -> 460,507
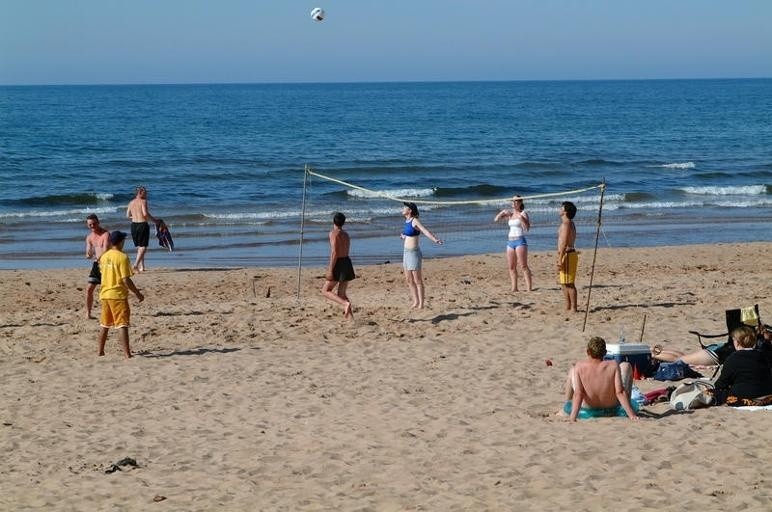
601,342 -> 651,377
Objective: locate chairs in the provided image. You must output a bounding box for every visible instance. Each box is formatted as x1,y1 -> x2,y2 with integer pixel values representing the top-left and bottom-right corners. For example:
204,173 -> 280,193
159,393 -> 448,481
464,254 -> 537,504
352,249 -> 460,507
690,305 -> 764,382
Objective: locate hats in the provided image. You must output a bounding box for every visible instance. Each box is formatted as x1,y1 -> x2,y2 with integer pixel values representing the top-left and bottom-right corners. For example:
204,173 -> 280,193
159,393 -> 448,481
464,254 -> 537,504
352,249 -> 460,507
110,231 -> 127,245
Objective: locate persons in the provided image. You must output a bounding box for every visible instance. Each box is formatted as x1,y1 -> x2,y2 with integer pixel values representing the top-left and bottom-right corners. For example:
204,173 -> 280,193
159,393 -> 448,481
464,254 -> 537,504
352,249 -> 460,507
96,230 -> 145,359
556,336 -> 641,423
493,194 -> 532,293
398,202 -> 443,311
126,185 -> 160,273
84,214 -> 113,320
713,326 -> 771,406
321,212 -> 356,321
651,324 -> 771,366
556,202 -> 579,313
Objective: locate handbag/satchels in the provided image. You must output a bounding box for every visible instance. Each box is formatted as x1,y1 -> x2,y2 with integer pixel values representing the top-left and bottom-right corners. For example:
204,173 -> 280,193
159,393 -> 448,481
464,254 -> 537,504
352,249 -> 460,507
656,360 -> 690,382
670,379 -> 716,411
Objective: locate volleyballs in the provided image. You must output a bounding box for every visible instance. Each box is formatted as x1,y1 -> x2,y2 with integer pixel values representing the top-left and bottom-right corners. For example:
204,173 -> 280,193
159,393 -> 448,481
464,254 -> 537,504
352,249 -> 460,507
311,7 -> 324,21
